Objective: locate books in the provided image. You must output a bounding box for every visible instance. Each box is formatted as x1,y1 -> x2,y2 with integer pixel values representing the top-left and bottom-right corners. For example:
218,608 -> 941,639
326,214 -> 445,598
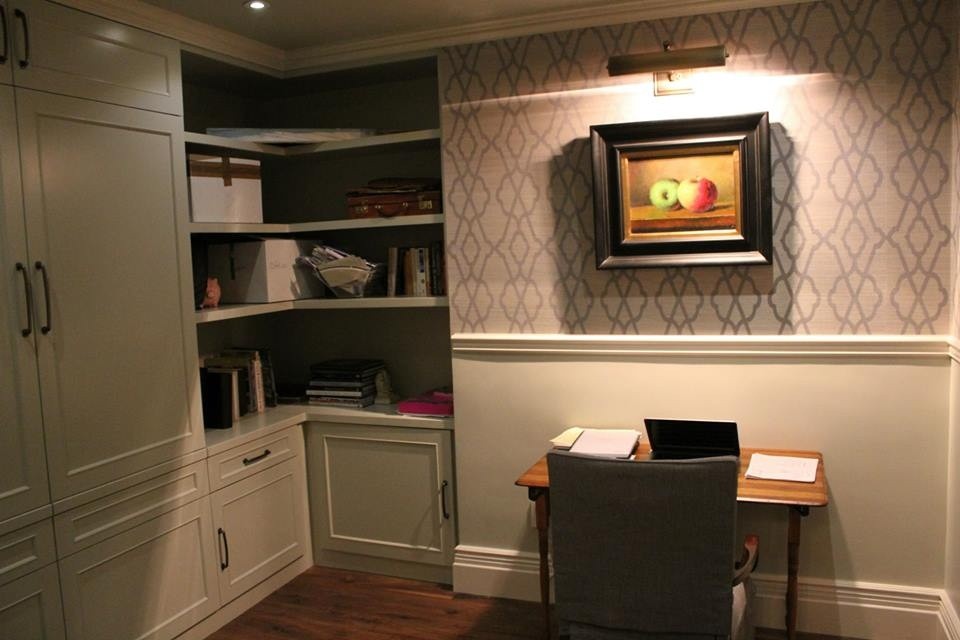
305,359 -> 387,408
551,428 -> 641,459
200,347 -> 278,430
386,241 -> 448,297
302,246 -> 385,295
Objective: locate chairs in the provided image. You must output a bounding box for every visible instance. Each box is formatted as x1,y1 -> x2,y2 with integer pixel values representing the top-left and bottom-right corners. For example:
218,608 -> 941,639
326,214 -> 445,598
548,451 -> 760,640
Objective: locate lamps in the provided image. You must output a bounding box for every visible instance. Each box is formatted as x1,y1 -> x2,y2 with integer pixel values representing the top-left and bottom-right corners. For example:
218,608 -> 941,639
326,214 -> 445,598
610,41 -> 731,96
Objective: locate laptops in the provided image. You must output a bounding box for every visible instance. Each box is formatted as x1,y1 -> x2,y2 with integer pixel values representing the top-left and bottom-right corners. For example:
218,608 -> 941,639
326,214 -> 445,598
645,418 -> 739,459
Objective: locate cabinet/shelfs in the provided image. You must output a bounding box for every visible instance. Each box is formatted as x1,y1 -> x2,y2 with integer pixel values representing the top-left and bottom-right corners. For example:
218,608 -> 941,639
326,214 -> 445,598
0,0 -> 457,640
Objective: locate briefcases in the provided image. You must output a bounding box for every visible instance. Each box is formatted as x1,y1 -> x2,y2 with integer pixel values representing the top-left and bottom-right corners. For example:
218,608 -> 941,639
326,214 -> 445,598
346,190 -> 441,220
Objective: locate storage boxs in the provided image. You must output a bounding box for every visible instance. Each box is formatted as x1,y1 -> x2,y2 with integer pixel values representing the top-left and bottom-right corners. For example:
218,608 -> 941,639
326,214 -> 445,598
186,155 -> 265,224
204,235 -> 327,304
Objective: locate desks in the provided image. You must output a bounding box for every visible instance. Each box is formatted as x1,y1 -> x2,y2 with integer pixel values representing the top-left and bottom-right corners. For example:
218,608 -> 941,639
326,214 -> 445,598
514,443 -> 828,640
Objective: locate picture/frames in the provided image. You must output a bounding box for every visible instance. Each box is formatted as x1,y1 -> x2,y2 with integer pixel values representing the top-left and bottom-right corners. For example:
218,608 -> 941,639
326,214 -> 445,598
589,111 -> 774,271
